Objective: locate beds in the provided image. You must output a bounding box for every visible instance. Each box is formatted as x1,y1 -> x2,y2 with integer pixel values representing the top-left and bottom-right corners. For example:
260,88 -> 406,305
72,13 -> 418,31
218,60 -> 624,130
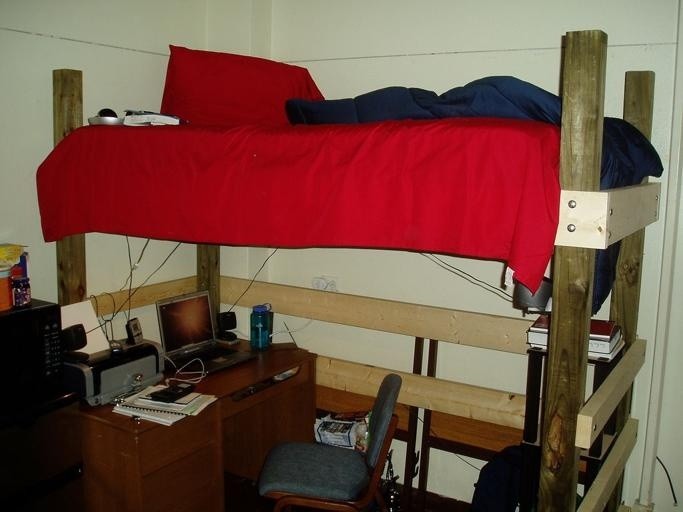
53,32 -> 662,511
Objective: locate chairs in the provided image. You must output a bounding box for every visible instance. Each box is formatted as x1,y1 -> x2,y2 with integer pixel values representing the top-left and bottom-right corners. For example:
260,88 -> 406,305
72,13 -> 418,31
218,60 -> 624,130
259,374 -> 403,511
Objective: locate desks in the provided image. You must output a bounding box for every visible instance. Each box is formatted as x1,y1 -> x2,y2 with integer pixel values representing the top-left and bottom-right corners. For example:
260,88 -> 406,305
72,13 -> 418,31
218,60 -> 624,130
34,337 -> 318,511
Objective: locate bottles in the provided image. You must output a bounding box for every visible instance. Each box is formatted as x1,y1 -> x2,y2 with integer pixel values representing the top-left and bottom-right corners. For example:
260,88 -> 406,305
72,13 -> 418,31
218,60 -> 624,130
250,302 -> 272,350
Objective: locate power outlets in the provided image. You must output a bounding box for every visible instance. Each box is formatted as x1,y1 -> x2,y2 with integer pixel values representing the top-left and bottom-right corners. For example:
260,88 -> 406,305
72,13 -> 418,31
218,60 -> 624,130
321,275 -> 336,291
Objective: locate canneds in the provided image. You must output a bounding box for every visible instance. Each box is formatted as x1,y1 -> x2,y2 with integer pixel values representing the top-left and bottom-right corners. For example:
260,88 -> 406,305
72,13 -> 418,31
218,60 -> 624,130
11,277 -> 31,307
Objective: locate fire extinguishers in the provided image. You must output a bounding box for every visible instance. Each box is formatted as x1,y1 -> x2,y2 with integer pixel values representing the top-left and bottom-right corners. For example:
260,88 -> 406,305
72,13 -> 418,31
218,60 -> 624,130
386,475 -> 401,512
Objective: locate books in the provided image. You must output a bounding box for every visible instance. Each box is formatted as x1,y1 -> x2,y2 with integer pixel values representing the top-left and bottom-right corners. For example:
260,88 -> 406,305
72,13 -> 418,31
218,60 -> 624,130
112,383 -> 218,427
525,311 -> 628,366
122,110 -> 180,127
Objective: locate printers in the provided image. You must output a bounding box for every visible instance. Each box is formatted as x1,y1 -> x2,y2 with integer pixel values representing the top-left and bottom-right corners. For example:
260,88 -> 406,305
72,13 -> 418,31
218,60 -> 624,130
63,336 -> 166,406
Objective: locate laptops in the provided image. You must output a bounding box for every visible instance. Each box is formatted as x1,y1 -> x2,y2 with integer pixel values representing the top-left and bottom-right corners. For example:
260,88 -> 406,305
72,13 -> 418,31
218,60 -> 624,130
155,289 -> 258,378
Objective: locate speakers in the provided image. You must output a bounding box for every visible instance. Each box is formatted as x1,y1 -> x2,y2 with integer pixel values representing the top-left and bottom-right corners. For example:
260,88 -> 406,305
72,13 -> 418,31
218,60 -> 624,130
63,323 -> 90,364
215,311 -> 237,340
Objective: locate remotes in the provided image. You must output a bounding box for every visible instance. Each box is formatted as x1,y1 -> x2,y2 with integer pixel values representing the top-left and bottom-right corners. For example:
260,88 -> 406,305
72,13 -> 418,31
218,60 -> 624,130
111,340 -> 123,353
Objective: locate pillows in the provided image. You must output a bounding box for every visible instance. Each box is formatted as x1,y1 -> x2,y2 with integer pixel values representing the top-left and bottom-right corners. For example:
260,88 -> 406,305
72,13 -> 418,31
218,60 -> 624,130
159,45 -> 326,128
